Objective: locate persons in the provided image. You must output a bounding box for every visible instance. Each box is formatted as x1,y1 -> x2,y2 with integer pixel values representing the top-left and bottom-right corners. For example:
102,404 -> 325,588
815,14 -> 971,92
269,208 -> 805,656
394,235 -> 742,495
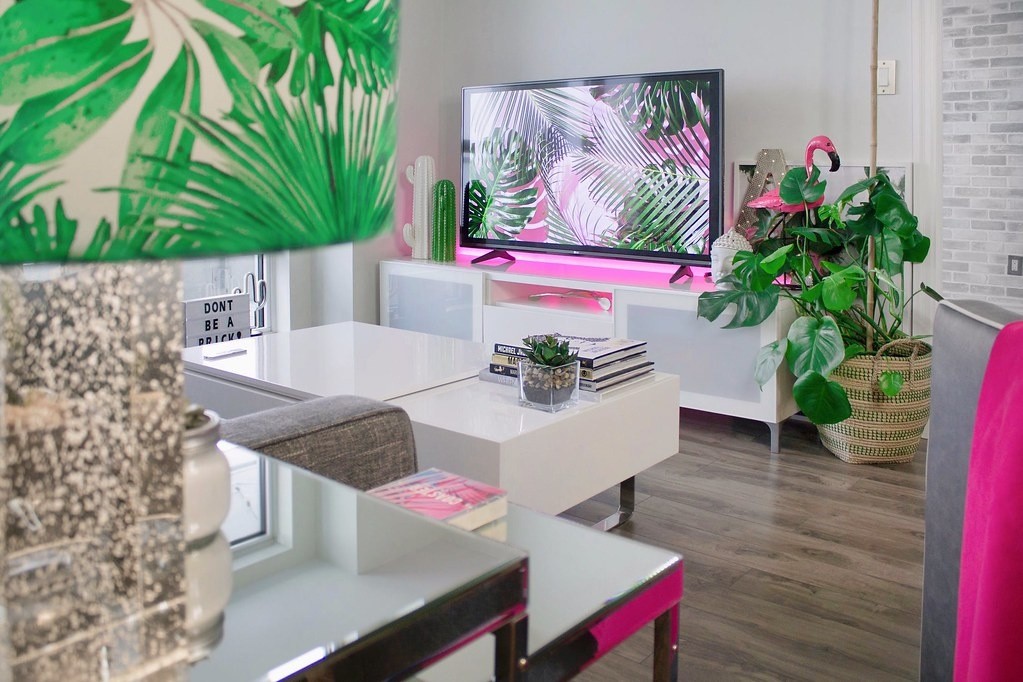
710,227 -> 753,290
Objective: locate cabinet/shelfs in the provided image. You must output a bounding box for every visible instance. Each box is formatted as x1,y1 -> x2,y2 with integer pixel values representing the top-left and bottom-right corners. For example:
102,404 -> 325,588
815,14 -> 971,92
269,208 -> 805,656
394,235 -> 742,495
379,257 -> 825,454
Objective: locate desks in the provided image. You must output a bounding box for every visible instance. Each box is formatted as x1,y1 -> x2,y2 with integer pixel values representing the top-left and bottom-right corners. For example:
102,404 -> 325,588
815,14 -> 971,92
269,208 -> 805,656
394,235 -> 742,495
182,438 -> 685,682
181,321 -> 680,533
184,438 -> 529,682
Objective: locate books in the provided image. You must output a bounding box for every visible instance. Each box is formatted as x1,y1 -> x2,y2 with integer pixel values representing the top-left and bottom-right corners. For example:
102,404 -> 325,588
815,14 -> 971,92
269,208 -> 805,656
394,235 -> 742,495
363,467 -> 508,543
479,333 -> 656,403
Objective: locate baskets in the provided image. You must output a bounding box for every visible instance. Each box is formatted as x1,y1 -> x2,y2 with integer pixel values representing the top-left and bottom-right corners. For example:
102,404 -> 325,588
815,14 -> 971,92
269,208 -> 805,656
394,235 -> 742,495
816,331 -> 934,465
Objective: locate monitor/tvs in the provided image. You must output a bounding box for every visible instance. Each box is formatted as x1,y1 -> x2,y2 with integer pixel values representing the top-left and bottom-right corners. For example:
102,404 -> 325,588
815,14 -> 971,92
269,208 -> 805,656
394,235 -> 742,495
459,69 -> 725,283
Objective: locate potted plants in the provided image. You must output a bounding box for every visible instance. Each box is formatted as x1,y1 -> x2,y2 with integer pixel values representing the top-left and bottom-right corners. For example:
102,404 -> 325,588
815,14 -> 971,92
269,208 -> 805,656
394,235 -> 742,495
697,163 -> 943,465
516,334 -> 581,414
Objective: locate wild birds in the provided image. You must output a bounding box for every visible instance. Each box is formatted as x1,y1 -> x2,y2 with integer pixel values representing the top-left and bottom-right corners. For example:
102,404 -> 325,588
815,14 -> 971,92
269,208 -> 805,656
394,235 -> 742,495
746,135 -> 841,292
